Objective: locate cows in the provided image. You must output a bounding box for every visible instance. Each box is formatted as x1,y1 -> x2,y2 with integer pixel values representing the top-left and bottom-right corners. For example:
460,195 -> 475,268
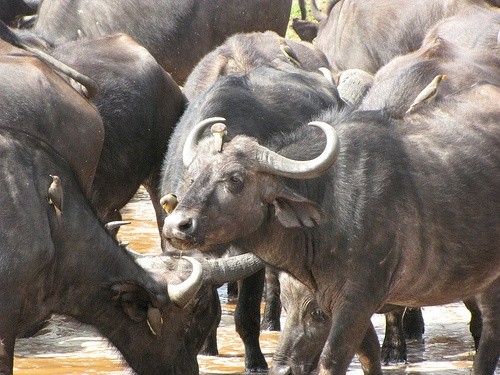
1,0 -> 500,375
